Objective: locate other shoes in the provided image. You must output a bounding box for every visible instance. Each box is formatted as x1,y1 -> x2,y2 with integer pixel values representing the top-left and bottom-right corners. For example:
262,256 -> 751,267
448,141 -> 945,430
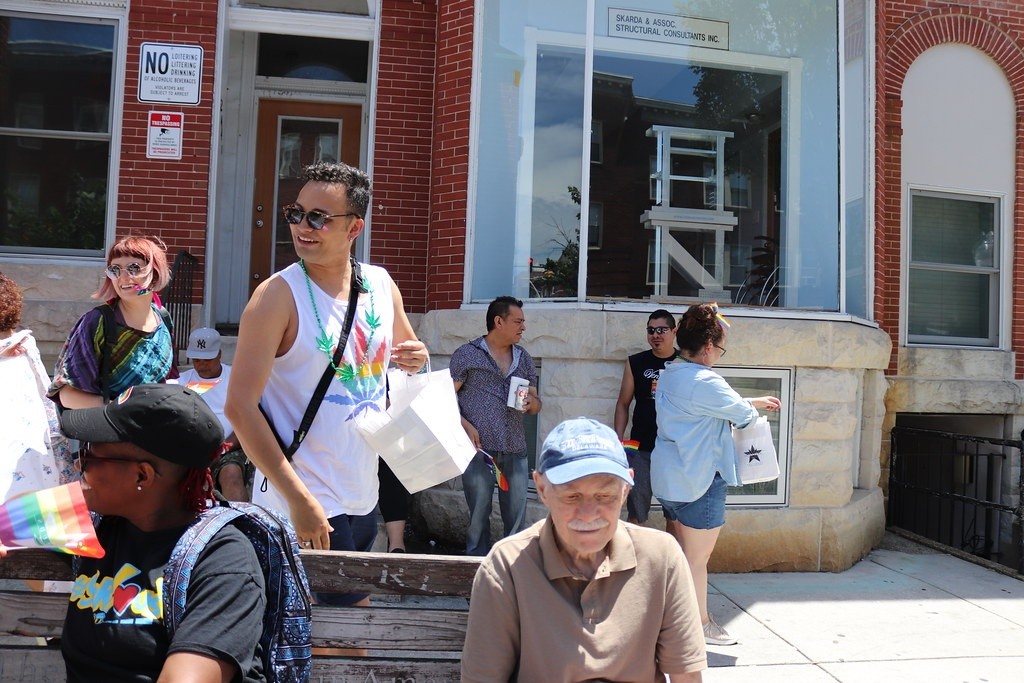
390,548 -> 404,553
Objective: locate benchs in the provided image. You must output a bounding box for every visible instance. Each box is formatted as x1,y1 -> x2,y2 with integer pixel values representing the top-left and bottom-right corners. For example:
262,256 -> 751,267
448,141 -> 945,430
0,549 -> 488,683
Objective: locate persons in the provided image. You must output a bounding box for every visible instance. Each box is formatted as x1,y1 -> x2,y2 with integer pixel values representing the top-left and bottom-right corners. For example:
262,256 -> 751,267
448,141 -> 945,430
650,304 -> 782,646
46,237 -> 180,581
0,384 -> 267,683
614,309 -> 679,539
451,296 -> 542,556
178,328 -> 257,503
0,271 -> 81,507
223,163 -> 428,607
378,369 -> 409,554
460,417 -> 707,683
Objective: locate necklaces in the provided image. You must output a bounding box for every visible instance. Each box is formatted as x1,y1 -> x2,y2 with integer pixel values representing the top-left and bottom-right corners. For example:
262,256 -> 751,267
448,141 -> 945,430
300,256 -> 381,382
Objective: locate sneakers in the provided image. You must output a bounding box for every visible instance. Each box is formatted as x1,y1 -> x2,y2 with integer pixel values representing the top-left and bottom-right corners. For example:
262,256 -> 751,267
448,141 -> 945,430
704,612 -> 738,645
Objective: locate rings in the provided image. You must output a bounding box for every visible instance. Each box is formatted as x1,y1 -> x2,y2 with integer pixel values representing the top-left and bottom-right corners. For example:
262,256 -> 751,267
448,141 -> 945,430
302,541 -> 311,547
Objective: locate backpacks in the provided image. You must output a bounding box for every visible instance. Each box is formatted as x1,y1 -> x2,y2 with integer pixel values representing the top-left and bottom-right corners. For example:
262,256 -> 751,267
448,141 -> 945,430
73,490 -> 312,683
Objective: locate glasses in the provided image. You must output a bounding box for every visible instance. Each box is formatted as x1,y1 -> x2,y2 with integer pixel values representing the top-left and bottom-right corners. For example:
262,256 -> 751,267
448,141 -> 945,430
105,263 -> 145,280
78,442 -> 160,476
646,326 -> 671,335
714,344 -> 727,358
283,204 -> 360,230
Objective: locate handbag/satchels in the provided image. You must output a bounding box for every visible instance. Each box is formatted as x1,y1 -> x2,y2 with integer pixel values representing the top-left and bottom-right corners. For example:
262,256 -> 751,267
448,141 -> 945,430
257,403 -> 294,463
354,341 -> 478,495
730,415 -> 780,485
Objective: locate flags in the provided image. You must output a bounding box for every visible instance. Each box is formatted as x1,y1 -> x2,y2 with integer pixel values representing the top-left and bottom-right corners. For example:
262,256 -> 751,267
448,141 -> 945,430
0,481 -> 105,558
484,454 -> 508,491
622,440 -> 640,455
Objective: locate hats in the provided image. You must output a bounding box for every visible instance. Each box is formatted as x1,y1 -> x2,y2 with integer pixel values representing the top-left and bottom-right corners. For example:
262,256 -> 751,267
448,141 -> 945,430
185,327 -> 221,360
536,416 -> 635,487
62,384 -> 224,470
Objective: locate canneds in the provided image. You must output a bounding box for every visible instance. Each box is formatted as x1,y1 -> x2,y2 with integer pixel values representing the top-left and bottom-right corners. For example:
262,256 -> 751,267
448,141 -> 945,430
515,384 -> 529,411
